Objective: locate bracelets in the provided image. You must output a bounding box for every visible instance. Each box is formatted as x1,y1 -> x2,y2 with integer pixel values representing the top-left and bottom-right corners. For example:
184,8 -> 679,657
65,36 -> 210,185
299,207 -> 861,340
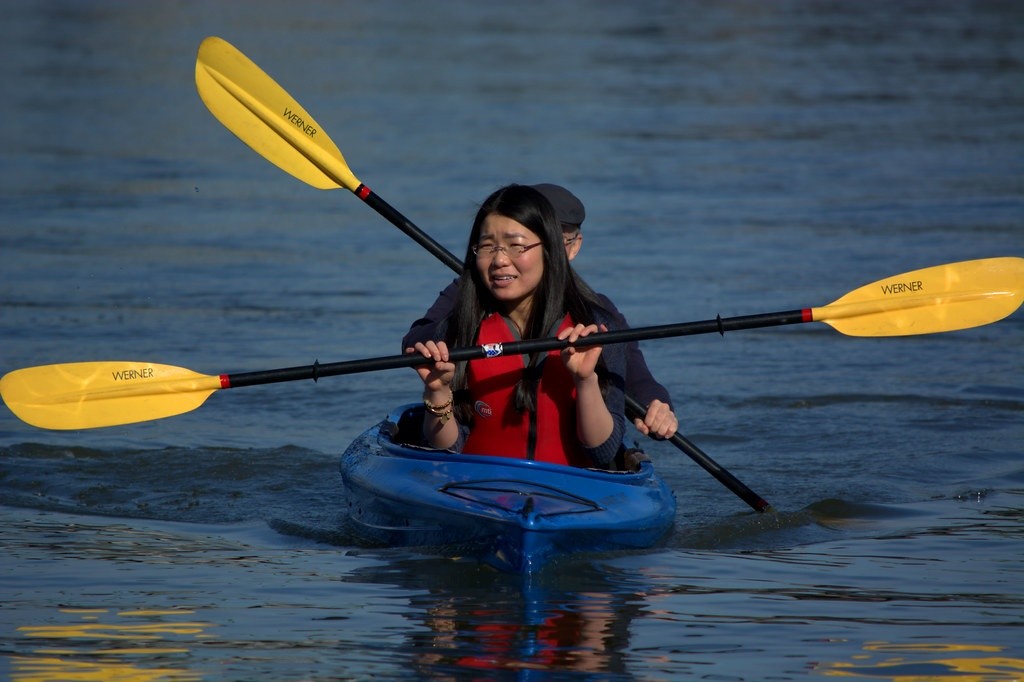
423,387 -> 454,423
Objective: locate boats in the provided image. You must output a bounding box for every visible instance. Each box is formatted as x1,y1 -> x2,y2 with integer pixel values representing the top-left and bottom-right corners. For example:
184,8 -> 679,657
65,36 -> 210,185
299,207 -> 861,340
336,408 -> 679,560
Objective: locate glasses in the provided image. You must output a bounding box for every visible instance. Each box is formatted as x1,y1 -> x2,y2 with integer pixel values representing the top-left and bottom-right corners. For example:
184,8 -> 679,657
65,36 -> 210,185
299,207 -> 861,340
473,240 -> 542,259
563,230 -> 578,247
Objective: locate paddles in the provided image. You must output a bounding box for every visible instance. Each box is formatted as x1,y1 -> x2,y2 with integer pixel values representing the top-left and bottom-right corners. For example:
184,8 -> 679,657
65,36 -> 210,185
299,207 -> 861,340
0,256 -> 1024,430
192,34 -> 774,517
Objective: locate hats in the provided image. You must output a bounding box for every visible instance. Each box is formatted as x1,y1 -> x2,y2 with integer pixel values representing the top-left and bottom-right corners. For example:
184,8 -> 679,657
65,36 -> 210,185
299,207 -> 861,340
530,182 -> 585,228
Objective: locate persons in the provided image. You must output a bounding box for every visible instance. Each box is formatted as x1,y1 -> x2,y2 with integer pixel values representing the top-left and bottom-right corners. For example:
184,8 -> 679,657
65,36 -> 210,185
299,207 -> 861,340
402,184 -> 678,472
